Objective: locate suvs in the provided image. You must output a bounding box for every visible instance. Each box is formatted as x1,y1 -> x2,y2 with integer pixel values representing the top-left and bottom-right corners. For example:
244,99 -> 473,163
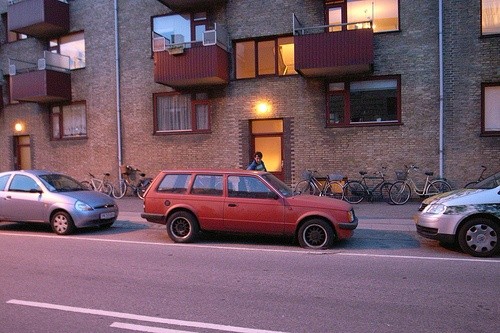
140,169 -> 358,249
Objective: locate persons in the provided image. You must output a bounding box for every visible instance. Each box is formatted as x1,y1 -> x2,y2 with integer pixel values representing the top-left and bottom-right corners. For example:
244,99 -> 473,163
245,152 -> 269,193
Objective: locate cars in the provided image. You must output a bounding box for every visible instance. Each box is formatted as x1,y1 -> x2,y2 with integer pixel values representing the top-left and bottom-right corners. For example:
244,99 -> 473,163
413,171 -> 500,257
0,170 -> 119,235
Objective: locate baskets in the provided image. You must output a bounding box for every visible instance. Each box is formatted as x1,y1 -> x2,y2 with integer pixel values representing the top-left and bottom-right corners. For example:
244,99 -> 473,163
396,171 -> 407,180
300,172 -> 309,179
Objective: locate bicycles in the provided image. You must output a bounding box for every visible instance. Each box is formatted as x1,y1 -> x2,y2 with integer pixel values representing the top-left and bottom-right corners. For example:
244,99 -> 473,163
464,165 -> 488,189
342,165 -> 402,205
388,164 -> 452,205
295,169 -> 344,201
112,165 -> 154,199
77,172 -> 112,197
